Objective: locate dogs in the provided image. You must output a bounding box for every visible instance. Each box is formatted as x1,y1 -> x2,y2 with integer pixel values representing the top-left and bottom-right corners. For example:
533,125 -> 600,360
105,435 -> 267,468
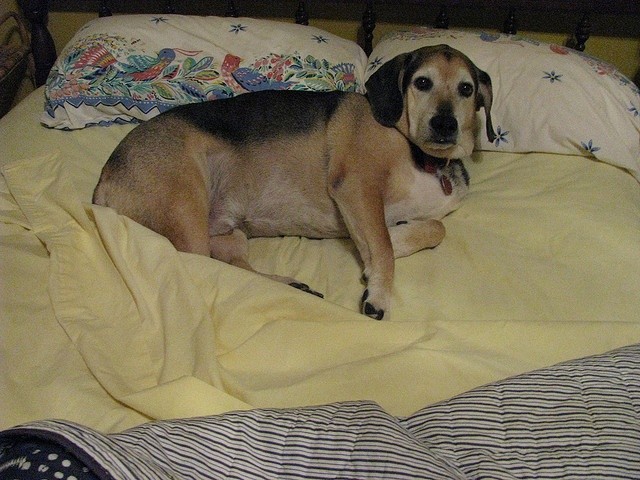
91,42 -> 499,323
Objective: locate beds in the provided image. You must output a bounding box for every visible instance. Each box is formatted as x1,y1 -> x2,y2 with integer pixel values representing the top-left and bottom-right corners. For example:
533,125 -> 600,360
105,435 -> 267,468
0,0 -> 640,480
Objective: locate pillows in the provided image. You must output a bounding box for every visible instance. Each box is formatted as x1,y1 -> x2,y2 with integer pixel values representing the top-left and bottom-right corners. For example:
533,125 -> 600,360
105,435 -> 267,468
39,12 -> 368,132
366,27 -> 639,183
